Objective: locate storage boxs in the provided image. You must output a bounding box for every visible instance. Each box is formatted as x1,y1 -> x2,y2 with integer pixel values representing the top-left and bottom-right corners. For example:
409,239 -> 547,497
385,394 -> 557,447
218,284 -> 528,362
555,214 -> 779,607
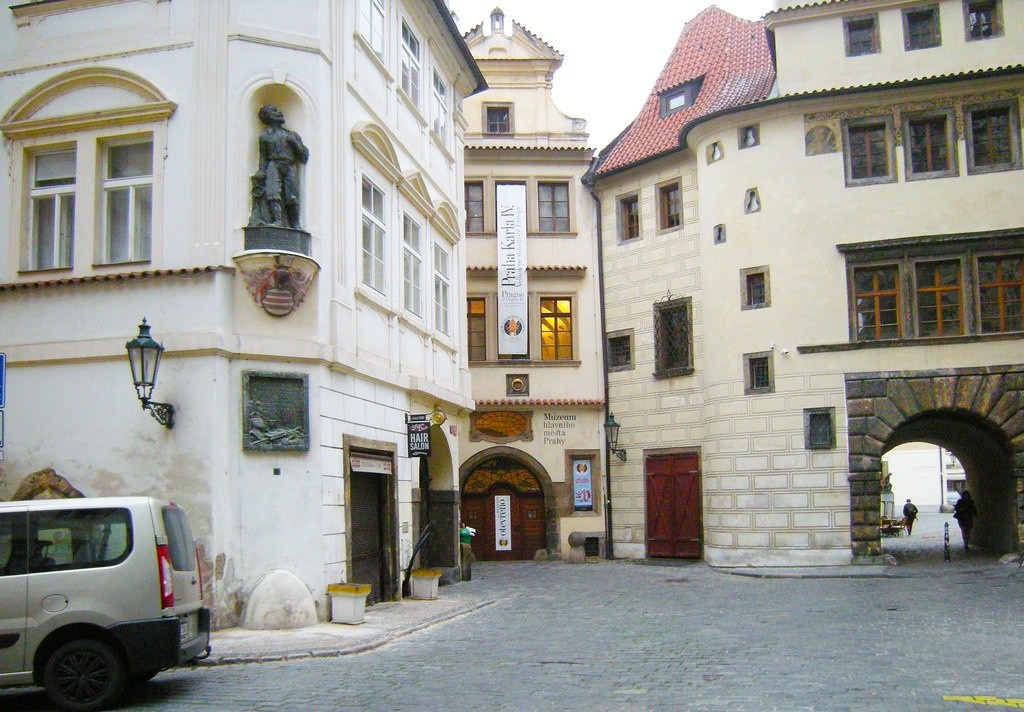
409,568 -> 442,600
327,583 -> 372,624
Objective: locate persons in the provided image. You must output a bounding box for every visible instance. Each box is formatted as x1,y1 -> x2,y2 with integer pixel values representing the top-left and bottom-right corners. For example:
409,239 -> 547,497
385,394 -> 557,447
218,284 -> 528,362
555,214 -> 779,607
954,490 -> 976,550
255,105 -> 310,232
903,498 -> 919,535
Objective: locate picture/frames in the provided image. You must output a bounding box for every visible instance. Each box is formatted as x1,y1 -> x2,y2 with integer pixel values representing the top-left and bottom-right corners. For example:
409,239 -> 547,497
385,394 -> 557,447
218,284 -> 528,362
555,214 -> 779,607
243,370 -> 310,451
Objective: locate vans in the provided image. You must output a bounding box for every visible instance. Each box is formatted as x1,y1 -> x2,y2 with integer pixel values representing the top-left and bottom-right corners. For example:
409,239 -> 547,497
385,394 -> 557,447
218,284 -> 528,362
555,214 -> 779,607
0,494 -> 213,711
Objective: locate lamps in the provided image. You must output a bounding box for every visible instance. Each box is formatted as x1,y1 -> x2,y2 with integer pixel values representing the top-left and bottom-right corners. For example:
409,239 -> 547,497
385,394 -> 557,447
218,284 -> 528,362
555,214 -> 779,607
603,411 -> 626,462
126,316 -> 174,430
